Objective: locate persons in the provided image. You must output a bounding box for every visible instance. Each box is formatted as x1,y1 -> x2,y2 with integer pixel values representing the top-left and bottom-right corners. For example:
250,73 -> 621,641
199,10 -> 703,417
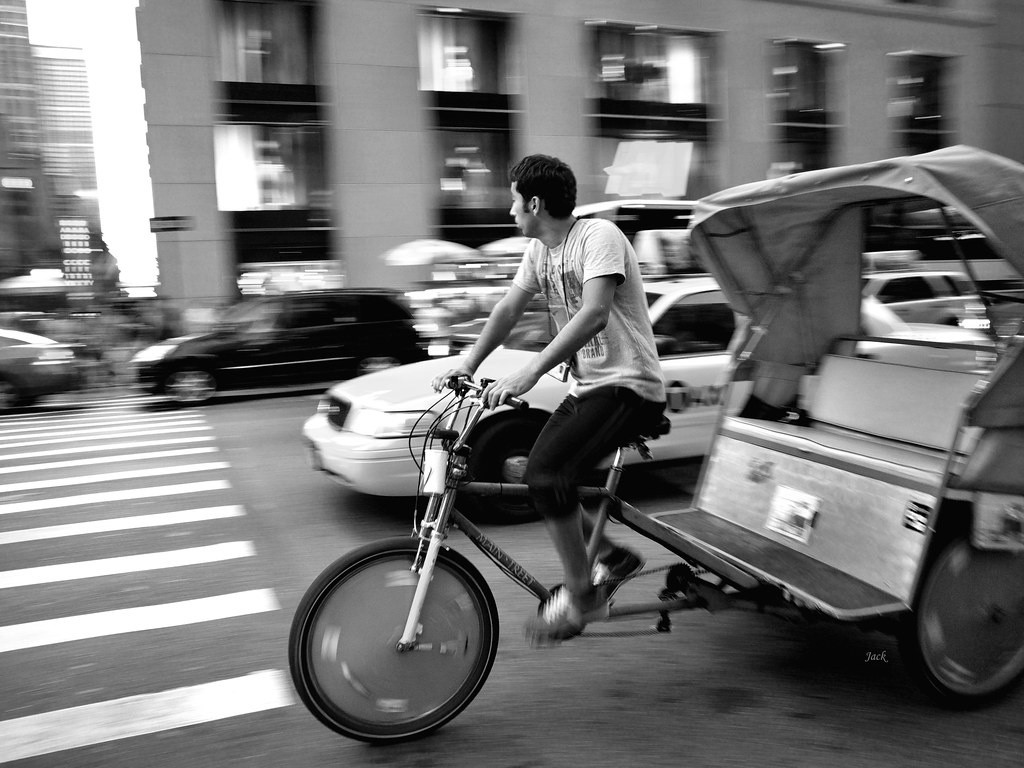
431,154 -> 668,643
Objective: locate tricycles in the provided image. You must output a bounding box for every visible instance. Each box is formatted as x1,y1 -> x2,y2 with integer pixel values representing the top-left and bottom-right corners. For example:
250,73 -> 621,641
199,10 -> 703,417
286,137 -> 1022,747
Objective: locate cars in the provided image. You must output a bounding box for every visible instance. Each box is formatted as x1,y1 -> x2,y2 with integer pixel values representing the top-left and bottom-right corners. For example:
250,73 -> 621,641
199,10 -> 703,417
0,329 -> 92,417
300,199 -> 1024,528
132,286 -> 435,411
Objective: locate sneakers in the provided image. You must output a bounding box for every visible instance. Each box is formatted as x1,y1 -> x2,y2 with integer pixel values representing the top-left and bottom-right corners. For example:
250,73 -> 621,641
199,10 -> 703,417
527,584 -> 608,640
585,549 -> 646,613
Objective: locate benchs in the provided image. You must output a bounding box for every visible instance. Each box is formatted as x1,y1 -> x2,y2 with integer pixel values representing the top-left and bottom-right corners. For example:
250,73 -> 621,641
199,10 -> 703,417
711,353 -> 988,500
